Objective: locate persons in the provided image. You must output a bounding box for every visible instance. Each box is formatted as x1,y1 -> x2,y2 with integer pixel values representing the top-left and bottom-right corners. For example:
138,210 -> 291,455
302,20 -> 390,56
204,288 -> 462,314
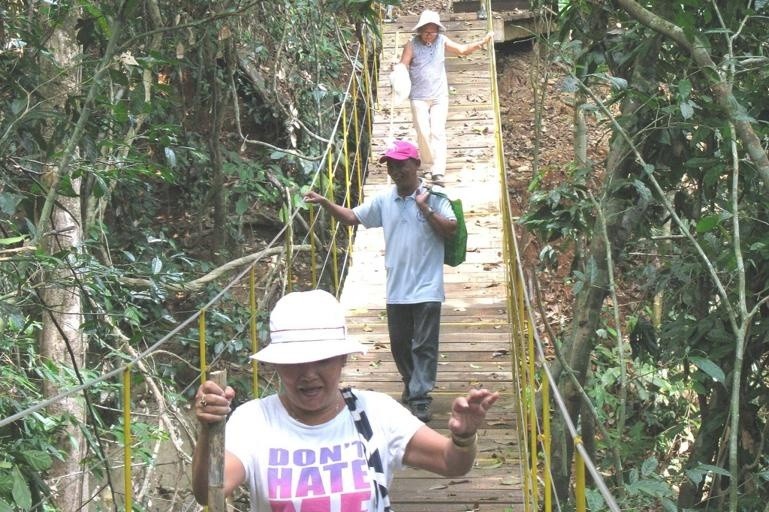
387,7 -> 496,188
187,288 -> 501,512
301,138 -> 459,425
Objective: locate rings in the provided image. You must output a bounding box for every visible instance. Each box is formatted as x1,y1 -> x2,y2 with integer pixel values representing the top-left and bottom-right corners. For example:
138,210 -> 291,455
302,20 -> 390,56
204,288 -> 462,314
199,394 -> 208,408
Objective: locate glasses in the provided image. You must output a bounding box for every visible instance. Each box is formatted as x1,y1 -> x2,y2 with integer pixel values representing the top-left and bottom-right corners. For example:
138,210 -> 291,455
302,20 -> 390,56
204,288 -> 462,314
423,32 -> 437,36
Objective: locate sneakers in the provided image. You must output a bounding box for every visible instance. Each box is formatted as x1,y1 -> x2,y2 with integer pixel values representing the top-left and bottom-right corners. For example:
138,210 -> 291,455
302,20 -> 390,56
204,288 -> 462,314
424,172 -> 446,187
402,388 -> 432,422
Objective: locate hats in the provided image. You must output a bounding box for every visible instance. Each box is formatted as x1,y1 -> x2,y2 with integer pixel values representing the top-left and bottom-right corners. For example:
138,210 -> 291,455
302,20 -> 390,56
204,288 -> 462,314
412,11 -> 446,32
249,290 -> 366,365
379,140 -> 421,166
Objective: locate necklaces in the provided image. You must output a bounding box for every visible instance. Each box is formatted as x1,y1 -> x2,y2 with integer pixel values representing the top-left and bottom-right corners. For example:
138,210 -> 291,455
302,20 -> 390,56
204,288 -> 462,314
285,391 -> 342,426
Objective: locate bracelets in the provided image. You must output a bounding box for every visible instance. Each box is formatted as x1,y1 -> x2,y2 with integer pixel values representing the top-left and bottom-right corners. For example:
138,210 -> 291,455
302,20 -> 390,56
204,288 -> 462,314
450,430 -> 477,443
421,206 -> 436,219
449,437 -> 478,454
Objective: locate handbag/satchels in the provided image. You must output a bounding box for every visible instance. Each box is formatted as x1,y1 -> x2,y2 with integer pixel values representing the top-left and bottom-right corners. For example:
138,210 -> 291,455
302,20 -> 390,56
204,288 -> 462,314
444,199 -> 467,267
